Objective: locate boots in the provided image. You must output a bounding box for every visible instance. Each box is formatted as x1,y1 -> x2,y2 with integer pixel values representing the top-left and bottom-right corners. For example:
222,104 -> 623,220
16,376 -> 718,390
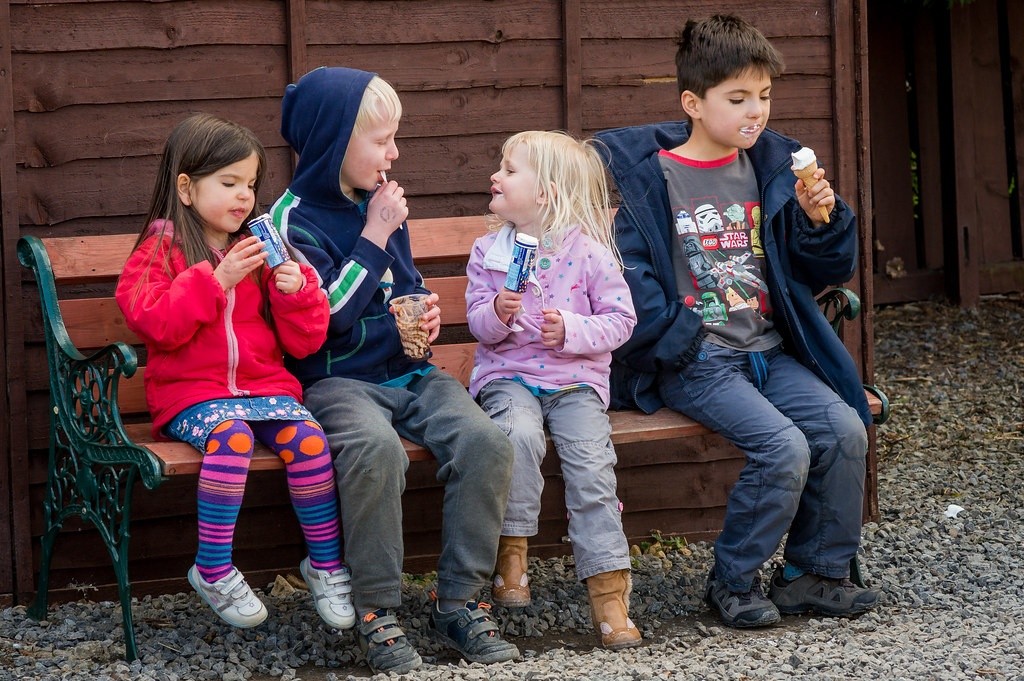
586,569 -> 643,650
491,535 -> 531,608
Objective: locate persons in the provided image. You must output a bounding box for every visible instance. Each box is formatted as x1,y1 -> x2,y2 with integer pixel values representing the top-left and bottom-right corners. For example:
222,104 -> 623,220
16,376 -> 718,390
270,67 -> 517,675
115,115 -> 356,629
465,130 -> 643,650
595,13 -> 888,629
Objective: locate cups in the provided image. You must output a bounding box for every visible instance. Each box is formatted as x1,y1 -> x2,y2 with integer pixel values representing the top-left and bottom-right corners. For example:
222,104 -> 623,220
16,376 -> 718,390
389,293 -> 433,362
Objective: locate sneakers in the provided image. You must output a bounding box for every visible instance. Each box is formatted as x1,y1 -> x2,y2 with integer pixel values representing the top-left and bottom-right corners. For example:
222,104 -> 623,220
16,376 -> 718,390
426,590 -> 520,664
352,608 -> 423,676
766,564 -> 882,620
187,563 -> 269,629
300,555 -> 356,630
702,565 -> 782,628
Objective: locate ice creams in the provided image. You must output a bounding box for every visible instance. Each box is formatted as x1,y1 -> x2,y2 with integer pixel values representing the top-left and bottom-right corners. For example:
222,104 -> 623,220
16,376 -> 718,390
791,147 -> 830,224
390,293 -> 432,361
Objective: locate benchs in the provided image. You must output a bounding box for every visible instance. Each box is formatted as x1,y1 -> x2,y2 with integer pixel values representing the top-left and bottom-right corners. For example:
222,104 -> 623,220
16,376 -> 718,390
14,212 -> 891,665
